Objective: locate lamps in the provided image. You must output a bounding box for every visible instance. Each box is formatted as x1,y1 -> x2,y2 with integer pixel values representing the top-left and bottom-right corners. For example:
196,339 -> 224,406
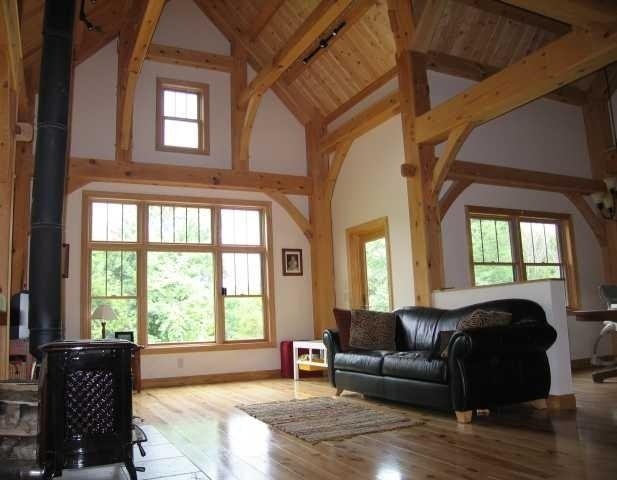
590,68 -> 617,220
88,305 -> 118,339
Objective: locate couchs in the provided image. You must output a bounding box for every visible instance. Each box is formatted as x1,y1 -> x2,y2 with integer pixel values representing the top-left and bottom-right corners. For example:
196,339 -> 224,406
323,298 -> 557,424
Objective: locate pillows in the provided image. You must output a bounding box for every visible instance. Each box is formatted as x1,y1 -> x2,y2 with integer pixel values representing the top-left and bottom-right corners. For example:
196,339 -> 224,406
427,309 -> 512,361
333,307 -> 397,352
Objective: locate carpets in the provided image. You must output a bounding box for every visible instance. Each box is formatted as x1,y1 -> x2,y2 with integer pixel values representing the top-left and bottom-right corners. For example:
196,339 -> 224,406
234,397 -> 424,445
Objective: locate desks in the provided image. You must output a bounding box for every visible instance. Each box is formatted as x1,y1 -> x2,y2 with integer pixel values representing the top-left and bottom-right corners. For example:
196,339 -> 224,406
293,340 -> 329,380
131,344 -> 144,393
572,309 -> 617,383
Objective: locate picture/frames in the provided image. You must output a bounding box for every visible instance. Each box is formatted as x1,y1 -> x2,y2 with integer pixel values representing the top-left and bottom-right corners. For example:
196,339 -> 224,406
115,331 -> 134,343
282,248 -> 303,276
